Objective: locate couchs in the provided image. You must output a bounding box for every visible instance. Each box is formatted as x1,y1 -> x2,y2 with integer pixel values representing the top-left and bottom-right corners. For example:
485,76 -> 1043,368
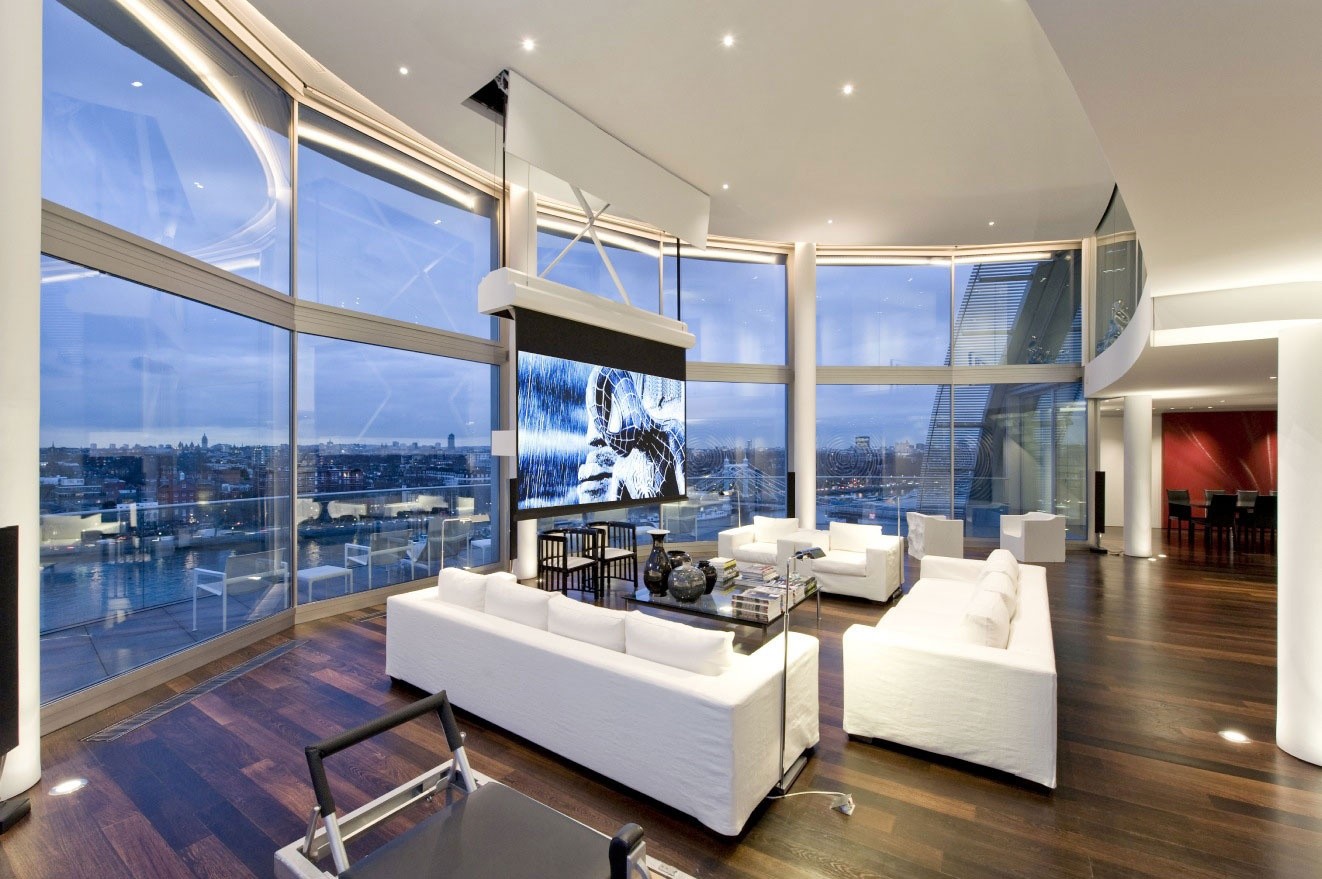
385,572 -> 820,836
1000,512 -> 1066,562
906,512 -> 964,560
843,554 -> 1059,789
718,524 -> 820,602
795,530 -> 905,602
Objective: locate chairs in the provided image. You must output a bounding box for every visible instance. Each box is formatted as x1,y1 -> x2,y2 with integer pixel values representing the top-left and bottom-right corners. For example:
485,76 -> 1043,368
345,516 -> 473,593
537,528 -> 599,600
192,548 -> 288,631
586,521 -> 639,597
1165,488 -> 1277,553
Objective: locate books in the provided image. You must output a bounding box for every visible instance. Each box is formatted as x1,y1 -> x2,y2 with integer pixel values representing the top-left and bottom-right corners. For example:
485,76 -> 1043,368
731,586 -> 793,624
707,558 -> 740,590
763,572 -> 818,604
735,564 -> 780,588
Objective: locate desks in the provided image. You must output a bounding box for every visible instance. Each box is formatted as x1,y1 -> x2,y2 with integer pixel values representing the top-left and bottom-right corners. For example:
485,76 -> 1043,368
470,539 -> 491,563
1179,500 -> 1255,548
298,566 -> 353,602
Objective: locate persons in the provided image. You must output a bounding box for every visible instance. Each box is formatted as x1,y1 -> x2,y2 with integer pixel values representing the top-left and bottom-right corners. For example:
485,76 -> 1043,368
586,366 -> 686,501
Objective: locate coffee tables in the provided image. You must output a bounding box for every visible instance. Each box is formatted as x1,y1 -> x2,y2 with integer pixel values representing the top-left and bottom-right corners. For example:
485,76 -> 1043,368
621,573 -> 820,649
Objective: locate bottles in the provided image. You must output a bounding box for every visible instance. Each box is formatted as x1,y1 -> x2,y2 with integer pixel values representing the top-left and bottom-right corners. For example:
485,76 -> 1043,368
696,560 -> 718,594
643,529 -> 672,598
666,550 -> 693,571
667,557 -> 706,603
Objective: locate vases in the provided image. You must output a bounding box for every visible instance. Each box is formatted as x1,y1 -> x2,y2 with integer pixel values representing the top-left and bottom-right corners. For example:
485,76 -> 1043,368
644,530 -> 717,604
